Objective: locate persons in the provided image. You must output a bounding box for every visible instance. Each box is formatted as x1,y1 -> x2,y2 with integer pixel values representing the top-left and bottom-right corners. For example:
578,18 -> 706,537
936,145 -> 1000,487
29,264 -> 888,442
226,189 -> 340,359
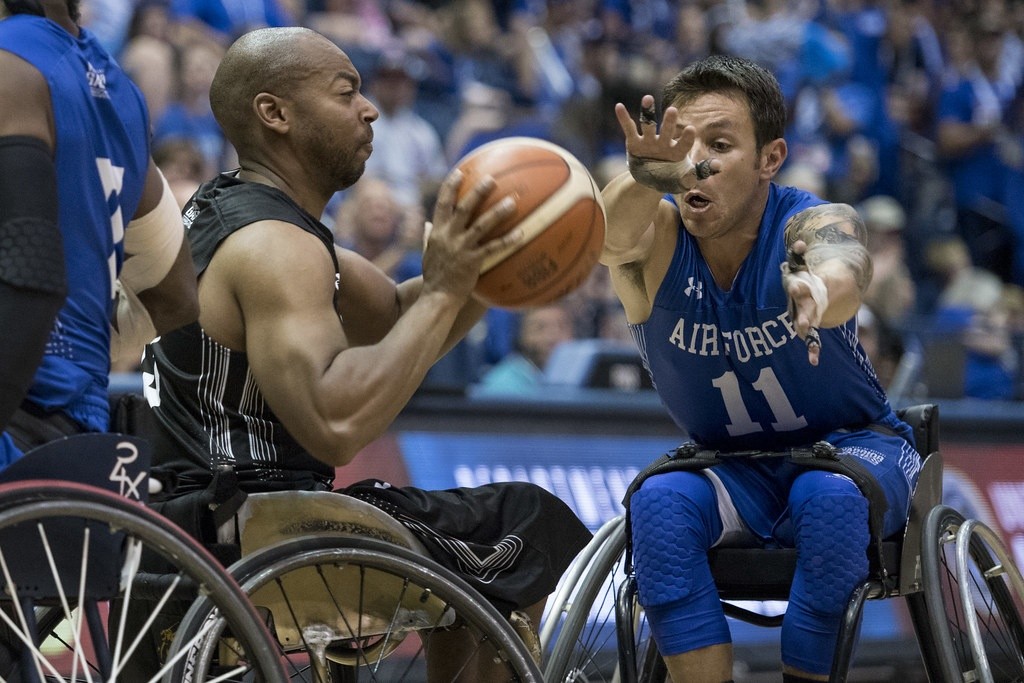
78,0 -> 1024,401
0,0 -> 204,683
139,26 -> 593,683
598,56 -> 922,683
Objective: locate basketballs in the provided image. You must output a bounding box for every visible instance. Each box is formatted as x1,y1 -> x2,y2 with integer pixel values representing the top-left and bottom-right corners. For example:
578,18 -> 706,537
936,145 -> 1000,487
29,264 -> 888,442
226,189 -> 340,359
427,131 -> 612,320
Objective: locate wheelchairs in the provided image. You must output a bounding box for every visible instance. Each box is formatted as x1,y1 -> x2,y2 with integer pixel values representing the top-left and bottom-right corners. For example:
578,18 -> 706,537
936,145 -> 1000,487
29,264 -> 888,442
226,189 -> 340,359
538,399 -> 1024,683
0,392 -> 539,682
